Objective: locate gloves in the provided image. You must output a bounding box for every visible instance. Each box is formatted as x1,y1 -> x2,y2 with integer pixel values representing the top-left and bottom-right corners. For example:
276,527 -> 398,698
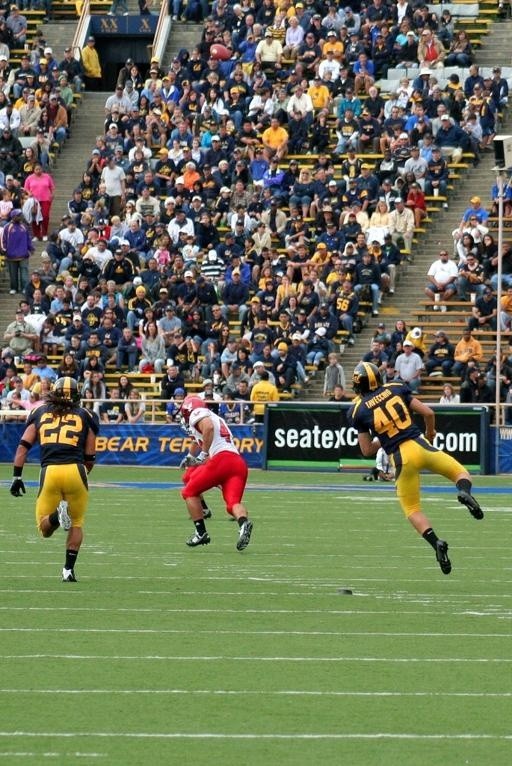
9,475 -> 26,498
195,451 -> 209,465
178,453 -> 195,469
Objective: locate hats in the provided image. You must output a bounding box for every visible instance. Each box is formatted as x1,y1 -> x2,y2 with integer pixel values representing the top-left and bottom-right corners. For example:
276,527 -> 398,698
0,0 -> 512,399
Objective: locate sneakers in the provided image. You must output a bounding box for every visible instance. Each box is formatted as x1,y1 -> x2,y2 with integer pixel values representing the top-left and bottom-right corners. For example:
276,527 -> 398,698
186,533 -> 211,547
437,540 -> 452,575
61,566 -> 77,583
457,492 -> 485,521
54,499 -> 71,530
237,521 -> 253,552
200,508 -> 212,520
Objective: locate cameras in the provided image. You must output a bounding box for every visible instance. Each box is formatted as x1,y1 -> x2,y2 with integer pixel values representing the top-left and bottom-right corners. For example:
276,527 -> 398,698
15,330 -> 21,337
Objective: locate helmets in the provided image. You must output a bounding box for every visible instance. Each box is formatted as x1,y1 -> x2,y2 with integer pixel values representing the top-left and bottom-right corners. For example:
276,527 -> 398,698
177,394 -> 208,422
53,376 -> 81,401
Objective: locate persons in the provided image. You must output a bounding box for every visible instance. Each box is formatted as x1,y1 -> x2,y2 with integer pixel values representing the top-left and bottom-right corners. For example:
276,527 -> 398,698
363,444 -> 396,483
10,374 -> 101,583
0,0 -> 512,424
345,358 -> 484,575
177,416 -> 248,523
173,395 -> 253,551
